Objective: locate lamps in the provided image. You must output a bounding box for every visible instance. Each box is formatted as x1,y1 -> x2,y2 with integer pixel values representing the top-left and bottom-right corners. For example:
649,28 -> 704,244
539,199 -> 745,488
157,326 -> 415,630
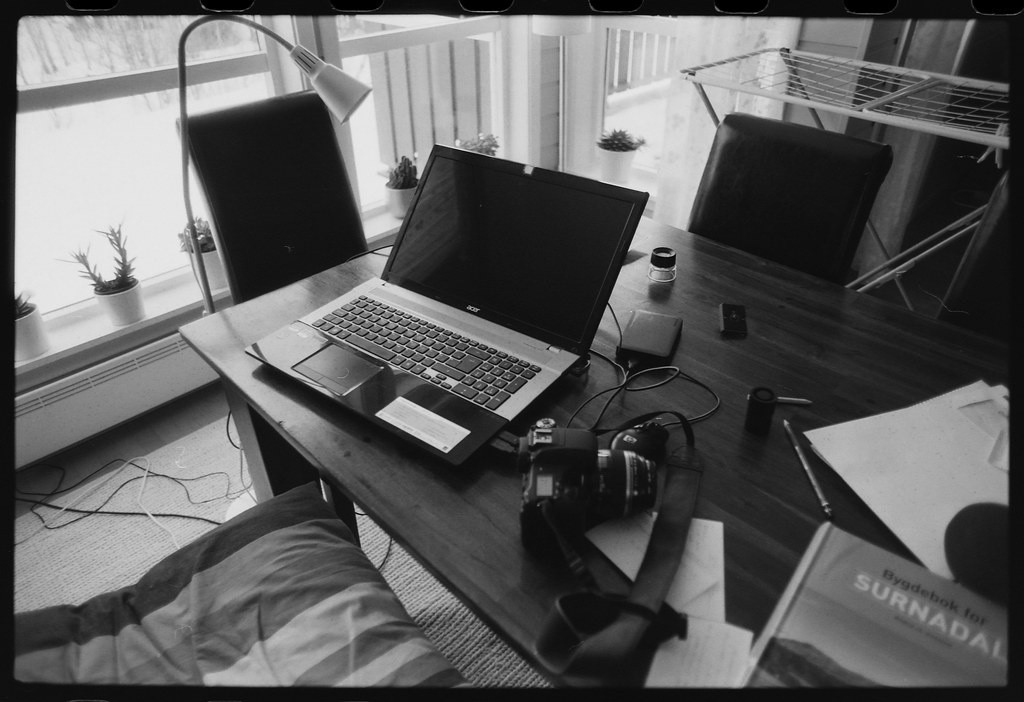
180,14 -> 372,317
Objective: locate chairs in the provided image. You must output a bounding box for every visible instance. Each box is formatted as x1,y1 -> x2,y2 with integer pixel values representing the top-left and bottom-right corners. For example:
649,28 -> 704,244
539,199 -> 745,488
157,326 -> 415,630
174,89 -> 369,548
14,480 -> 472,687
685,112 -> 894,287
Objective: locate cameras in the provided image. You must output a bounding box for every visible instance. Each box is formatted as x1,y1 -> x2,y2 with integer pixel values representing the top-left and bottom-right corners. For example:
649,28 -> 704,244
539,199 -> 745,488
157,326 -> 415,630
518,416 -> 656,564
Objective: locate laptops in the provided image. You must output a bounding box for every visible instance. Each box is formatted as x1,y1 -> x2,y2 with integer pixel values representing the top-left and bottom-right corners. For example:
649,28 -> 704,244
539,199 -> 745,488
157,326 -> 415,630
245,144 -> 649,465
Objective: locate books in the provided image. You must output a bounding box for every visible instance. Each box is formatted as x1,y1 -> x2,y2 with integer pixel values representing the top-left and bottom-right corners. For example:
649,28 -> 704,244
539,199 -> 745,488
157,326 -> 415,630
740,522 -> 1007,687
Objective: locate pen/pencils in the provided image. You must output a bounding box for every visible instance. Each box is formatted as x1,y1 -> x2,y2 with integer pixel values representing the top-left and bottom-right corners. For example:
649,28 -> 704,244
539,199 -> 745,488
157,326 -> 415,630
747,394 -> 813,404
783,419 -> 833,517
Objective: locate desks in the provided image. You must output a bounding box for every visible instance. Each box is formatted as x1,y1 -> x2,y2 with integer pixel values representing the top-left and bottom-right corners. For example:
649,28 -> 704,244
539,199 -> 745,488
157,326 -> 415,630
226,291 -> 1009,687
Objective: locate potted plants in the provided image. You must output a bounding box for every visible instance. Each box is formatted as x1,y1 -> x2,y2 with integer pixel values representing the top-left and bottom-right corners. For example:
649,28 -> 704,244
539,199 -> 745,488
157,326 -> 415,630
15,291 -> 51,362
384,154 -> 419,220
594,127 -> 646,187
54,222 -> 146,328
